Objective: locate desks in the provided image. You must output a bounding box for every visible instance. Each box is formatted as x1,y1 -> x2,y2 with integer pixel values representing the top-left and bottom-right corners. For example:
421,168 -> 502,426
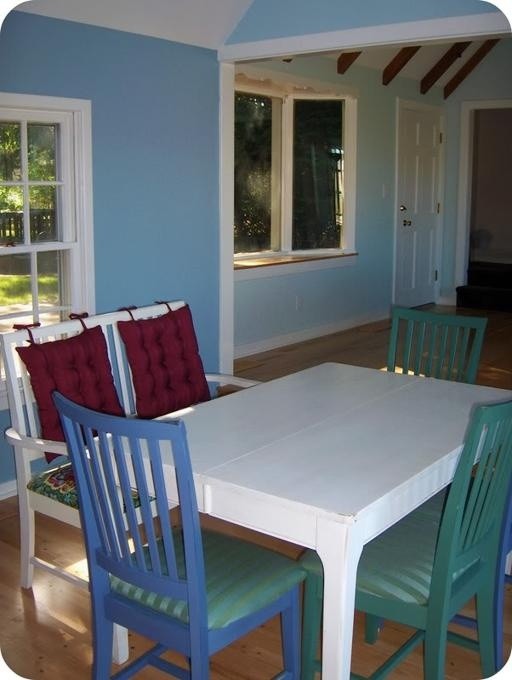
90,360 -> 512,680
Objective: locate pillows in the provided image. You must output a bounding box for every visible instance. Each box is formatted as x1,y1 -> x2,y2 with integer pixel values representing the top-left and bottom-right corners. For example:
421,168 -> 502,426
117,305 -> 213,419
15,325 -> 126,463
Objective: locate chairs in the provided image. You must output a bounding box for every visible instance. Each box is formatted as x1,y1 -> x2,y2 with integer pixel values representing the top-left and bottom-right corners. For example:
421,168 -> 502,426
53,388 -> 308,680
296,398 -> 512,680
376,478 -> 512,673
387,306 -> 487,385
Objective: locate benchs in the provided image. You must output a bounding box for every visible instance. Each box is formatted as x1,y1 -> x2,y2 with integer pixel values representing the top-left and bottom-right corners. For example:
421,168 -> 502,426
0,300 -> 266,666
